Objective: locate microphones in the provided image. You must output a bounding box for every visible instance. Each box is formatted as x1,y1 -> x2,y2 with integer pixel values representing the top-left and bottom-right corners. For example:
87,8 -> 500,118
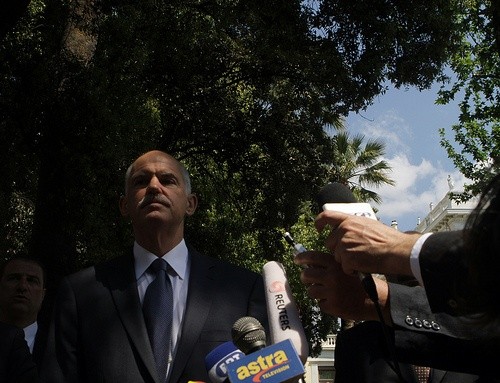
316,183 -> 379,303
263,261 -> 309,383
226,316 -> 306,383
205,341 -> 246,383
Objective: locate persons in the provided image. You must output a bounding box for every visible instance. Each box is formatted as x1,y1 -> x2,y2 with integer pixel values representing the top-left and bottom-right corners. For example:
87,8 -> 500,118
0,322 -> 39,383
39,149 -> 270,383
0,257 -> 50,355
295,175 -> 500,383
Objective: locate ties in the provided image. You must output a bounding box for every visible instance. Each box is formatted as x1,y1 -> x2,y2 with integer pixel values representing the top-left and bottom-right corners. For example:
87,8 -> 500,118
141,259 -> 178,382
417,367 -> 430,383
